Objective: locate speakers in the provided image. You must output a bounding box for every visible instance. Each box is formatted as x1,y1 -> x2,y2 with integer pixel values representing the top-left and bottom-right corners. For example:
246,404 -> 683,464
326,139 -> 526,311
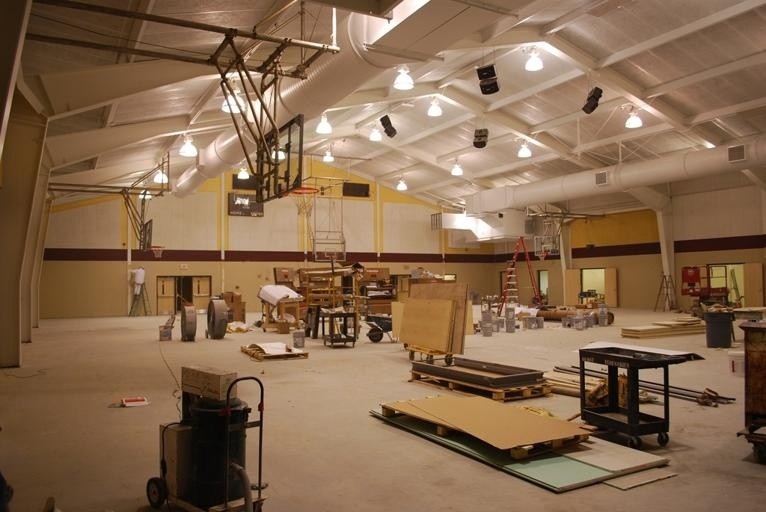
477,63 -> 497,80
379,114 -> 391,127
582,97 -> 599,114
472,136 -> 488,148
384,125 -> 397,138
586,86 -> 602,101
478,77 -> 500,95
474,127 -> 489,138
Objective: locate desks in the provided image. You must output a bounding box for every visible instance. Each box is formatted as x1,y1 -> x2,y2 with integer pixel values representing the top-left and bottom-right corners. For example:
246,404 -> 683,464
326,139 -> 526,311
575,344 -> 685,448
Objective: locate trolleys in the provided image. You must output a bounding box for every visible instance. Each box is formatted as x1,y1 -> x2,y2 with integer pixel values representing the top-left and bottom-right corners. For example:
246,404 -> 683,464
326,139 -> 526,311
365,315 -> 393,341
144,376 -> 266,512
578,340 -> 702,449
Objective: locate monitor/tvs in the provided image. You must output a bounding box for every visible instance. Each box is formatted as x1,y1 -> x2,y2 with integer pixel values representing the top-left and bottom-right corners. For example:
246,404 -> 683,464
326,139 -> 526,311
343,182 -> 369,197
227,192 -> 265,218
233,174 -> 263,190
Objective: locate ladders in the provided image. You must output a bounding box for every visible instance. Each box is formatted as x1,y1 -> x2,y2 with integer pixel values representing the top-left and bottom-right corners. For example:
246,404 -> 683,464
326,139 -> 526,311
129,282 -> 152,317
654,274 -> 678,312
497,237 -> 541,317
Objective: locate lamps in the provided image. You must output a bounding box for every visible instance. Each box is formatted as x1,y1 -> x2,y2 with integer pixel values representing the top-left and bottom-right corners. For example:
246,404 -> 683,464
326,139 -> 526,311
395,169 -> 408,191
451,156 -> 463,175
476,46 -> 500,96
524,48 -> 544,72
583,73 -> 603,116
473,110 -> 489,148
139,83 -> 245,200
517,138 -> 533,158
624,104 -> 643,128
270,61 -> 445,162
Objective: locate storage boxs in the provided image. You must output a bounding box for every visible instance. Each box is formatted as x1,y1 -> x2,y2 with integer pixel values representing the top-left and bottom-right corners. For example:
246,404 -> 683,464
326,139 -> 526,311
154,363 -> 254,512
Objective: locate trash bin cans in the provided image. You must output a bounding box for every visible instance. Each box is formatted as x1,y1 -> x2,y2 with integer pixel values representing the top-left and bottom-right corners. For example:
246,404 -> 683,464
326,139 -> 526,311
704,312 -> 736,348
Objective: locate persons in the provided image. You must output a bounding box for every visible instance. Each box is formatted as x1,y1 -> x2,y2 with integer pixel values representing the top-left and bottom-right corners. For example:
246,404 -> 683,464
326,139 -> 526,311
336,299 -> 362,341
